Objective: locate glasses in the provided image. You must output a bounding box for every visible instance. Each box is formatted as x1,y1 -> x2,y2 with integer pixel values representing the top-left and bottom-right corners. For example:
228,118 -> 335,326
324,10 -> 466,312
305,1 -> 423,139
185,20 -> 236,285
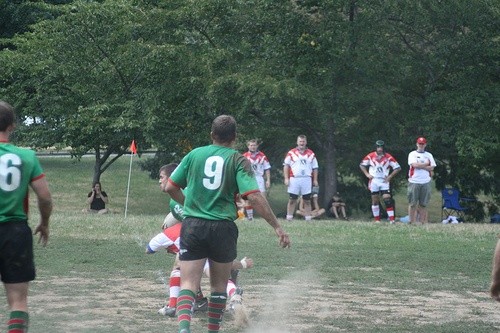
376,145 -> 383,148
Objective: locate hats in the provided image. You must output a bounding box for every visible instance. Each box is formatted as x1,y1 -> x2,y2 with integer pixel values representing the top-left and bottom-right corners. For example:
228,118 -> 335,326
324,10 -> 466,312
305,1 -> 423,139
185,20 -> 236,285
334,191 -> 340,196
417,137 -> 426,144
376,141 -> 384,146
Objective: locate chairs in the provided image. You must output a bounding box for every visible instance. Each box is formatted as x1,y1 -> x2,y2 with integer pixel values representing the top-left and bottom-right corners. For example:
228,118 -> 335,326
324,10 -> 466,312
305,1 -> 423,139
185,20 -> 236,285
440,187 -> 476,223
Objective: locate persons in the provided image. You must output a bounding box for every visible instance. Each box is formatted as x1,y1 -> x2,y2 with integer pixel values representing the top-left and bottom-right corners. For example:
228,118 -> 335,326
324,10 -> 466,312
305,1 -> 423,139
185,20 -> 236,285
165,115 -> 291,333
0,100 -> 53,333
283,135 -> 319,221
242,138 -> 271,220
327,192 -> 348,220
296,193 -> 326,220
146,163 -> 254,333
407,137 -> 437,225
82,182 -> 109,216
360,140 -> 402,224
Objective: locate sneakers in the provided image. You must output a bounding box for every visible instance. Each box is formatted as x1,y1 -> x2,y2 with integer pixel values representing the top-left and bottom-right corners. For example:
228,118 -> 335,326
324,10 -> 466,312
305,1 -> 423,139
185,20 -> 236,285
158,306 -> 176,316
192,301 -> 208,310
344,216 -> 349,220
230,295 -> 243,310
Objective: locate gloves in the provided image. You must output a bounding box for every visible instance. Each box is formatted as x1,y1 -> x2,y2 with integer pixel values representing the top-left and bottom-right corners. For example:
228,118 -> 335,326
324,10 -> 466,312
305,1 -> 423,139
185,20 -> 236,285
241,257 -> 253,269
